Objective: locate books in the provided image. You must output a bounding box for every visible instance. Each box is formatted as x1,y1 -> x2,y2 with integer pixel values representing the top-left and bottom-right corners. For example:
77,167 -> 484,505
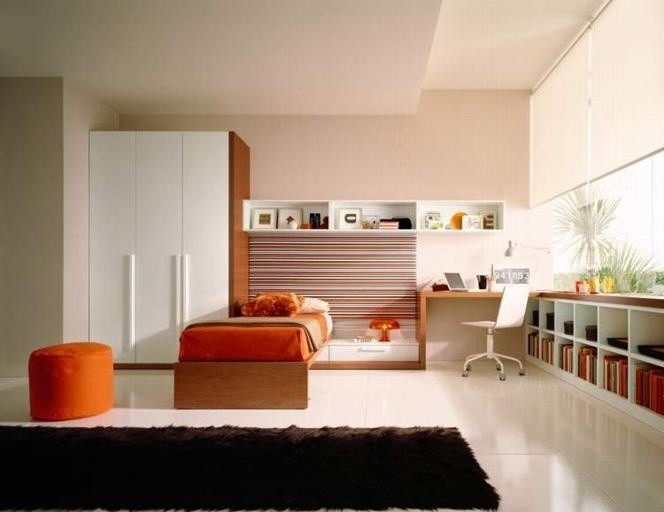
602,355 -> 627,399
528,332 -> 538,357
633,363 -> 664,414
541,337 -> 553,364
562,343 -> 573,373
577,344 -> 597,385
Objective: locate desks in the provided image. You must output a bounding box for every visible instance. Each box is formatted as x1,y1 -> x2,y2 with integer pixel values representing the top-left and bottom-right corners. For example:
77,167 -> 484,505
420,291 -> 535,371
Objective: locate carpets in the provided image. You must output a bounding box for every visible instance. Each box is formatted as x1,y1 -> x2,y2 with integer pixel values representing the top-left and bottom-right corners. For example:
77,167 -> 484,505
0,425 -> 501,511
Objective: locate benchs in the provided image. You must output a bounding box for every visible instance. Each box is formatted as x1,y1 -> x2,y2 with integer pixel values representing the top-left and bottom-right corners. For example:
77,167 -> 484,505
173,314 -> 333,410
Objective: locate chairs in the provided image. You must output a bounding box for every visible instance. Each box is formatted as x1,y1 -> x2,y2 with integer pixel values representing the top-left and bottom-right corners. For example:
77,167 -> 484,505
461,284 -> 530,381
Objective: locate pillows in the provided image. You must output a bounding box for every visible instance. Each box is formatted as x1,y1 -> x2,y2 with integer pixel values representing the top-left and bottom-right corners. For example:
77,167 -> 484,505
240,289 -> 306,318
297,296 -> 331,315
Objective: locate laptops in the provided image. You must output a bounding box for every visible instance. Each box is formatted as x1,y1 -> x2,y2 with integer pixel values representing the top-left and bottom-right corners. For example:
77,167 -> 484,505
443,271 -> 470,292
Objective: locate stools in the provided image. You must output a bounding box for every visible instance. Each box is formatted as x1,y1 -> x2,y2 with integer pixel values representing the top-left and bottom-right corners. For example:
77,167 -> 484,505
28,342 -> 114,423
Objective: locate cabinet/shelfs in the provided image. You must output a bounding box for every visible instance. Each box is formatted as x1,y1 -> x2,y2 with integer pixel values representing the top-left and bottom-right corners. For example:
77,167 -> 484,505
242,199 -> 506,237
89,131 -> 135,369
135,131 -> 250,370
525,296 -> 664,433
